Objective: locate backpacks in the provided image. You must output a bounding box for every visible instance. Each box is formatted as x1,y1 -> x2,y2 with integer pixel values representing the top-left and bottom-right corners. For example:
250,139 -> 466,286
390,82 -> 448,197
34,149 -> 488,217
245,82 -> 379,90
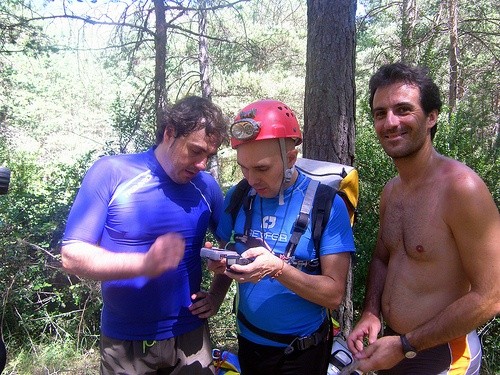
224,155 -> 359,268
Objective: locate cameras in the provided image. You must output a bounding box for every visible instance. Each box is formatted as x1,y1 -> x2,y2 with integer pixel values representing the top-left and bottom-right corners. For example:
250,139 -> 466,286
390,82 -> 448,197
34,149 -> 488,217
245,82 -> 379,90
199,247 -> 237,262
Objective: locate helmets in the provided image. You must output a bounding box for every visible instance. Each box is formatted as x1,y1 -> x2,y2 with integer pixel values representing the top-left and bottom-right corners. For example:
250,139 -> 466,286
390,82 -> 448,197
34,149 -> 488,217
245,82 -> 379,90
229,99 -> 303,149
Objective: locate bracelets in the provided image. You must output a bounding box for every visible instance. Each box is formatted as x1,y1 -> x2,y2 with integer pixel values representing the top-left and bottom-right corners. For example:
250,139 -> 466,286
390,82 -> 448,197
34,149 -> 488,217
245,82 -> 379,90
271,260 -> 285,279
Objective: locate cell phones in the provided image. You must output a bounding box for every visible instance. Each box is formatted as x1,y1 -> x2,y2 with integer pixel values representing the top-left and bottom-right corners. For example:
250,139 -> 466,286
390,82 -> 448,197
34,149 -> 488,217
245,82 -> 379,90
226,255 -> 254,270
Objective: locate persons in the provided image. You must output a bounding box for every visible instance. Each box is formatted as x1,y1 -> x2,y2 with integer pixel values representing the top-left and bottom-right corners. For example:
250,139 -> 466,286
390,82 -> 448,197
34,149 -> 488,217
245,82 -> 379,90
60,94 -> 234,375
347,64 -> 500,375
204,100 -> 356,375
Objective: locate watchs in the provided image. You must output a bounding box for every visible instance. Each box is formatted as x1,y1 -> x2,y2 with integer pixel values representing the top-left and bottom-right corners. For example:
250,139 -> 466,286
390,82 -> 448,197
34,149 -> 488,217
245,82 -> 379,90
400,334 -> 417,360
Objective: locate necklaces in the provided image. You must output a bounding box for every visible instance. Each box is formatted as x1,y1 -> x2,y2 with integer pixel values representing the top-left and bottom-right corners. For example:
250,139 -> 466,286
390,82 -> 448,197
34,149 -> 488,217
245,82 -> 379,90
260,173 -> 299,254
